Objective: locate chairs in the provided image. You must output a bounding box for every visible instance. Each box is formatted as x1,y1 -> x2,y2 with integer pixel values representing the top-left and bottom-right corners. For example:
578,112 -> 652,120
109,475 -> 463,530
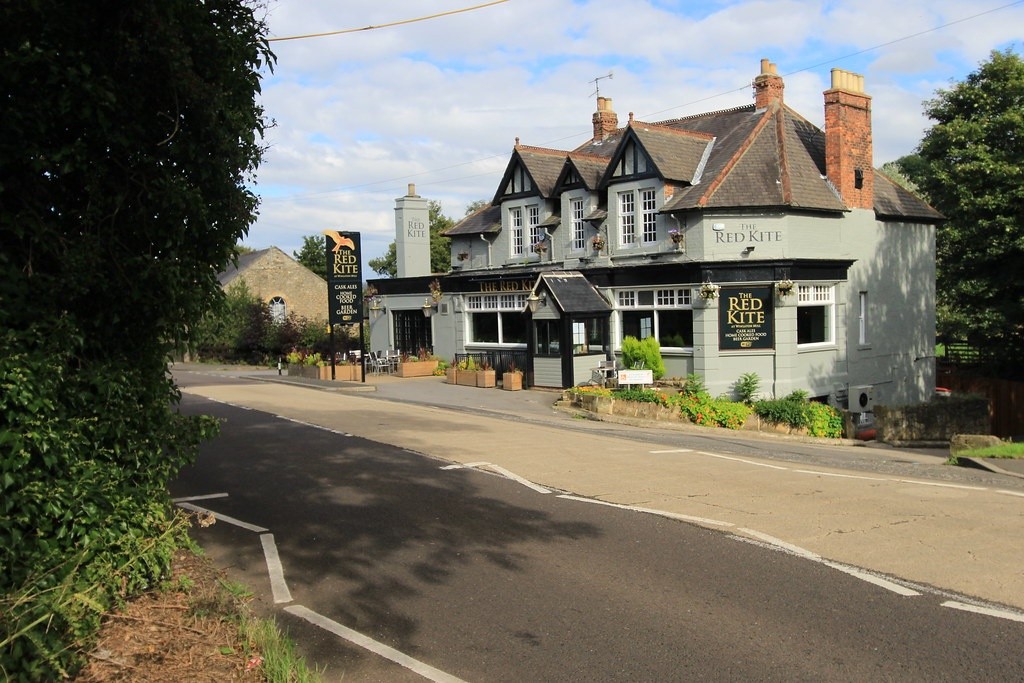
586,361 -> 647,389
348,349 -> 402,376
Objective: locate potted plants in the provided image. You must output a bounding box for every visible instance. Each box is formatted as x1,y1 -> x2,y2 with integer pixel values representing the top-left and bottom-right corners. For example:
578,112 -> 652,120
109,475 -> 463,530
397,350 -> 439,377
457,358 -> 476,386
504,361 -> 522,391
477,363 -> 497,388
777,283 -> 796,296
446,357 -> 456,384
701,285 -> 721,300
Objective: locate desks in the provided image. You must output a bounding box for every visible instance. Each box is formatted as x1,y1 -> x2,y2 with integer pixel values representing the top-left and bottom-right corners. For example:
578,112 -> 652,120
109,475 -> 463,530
362,354 -> 371,375
590,366 -> 618,389
382,354 -> 402,375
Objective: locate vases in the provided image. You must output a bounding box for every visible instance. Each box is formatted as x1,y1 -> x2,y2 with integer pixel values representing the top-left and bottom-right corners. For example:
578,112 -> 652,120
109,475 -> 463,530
433,300 -> 439,305
536,248 -> 548,252
593,242 -> 605,248
672,236 -> 684,243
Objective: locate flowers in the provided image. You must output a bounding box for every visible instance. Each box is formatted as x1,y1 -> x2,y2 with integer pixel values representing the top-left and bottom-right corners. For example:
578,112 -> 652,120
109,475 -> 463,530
669,228 -> 683,238
429,282 -> 443,302
534,242 -> 547,250
593,236 -> 604,244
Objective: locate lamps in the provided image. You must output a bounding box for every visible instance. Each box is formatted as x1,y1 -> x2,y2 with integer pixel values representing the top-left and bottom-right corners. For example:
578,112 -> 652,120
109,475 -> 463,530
422,297 -> 432,318
744,246 -> 757,254
370,306 -> 387,318
527,290 -> 547,312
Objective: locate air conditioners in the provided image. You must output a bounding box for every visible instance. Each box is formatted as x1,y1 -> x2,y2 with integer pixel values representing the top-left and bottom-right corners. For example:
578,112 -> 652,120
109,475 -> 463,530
848,384 -> 874,414
